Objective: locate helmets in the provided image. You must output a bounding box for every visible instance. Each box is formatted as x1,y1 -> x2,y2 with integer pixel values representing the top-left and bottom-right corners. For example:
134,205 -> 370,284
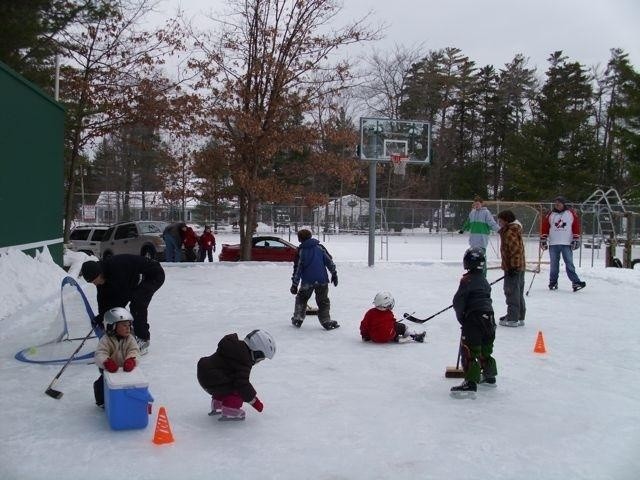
463,247 -> 486,270
372,290 -> 395,310
243,329 -> 276,363
104,307 -> 135,336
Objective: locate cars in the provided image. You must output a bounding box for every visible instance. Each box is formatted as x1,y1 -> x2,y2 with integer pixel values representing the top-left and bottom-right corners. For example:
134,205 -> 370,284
217,236 -> 299,262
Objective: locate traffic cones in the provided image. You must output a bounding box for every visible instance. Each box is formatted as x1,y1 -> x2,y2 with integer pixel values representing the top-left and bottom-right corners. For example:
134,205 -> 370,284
152,406 -> 175,445
533,331 -> 547,354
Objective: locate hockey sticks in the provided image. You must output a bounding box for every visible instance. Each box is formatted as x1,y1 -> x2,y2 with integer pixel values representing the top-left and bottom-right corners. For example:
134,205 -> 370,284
404,271 -> 505,324
525,244 -> 545,295
46,320 -> 102,400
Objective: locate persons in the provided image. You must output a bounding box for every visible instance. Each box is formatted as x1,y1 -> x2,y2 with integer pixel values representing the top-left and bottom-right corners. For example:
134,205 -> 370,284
497,210 -> 527,327
541,195 -> 586,292
198,223 -> 216,262
360,291 -> 429,346
196,327 -> 277,421
92,306 -> 141,409
162,221 -> 186,262
449,245 -> 498,394
181,226 -> 198,262
459,196 -> 501,279
288,229 -> 340,331
79,253 -> 166,357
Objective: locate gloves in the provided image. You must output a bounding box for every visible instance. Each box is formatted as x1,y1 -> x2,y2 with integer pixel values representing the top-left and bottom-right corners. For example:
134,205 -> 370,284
331,271 -> 338,287
290,286 -> 297,294
508,268 -> 517,277
124,357 -> 136,371
251,397 -> 263,411
571,234 -> 580,250
91,314 -> 104,328
104,356 -> 119,372
540,234 -> 548,250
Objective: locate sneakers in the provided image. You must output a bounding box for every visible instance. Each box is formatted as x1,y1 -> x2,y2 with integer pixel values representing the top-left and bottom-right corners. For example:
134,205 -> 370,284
549,280 -> 557,288
291,317 -> 303,327
95,400 -> 104,408
323,320 -> 337,330
212,398 -> 245,419
480,373 -> 496,383
572,281 -> 585,289
500,316 -> 517,322
450,379 -> 477,393
136,337 -> 150,352
415,331 -> 426,342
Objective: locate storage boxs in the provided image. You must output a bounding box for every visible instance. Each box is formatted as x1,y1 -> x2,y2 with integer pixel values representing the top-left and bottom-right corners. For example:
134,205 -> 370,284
103,367 -> 152,431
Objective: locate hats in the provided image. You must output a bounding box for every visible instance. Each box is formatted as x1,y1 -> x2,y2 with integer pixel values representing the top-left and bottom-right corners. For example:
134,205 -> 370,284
205,226 -> 212,233
81,261 -> 101,283
298,229 -> 312,241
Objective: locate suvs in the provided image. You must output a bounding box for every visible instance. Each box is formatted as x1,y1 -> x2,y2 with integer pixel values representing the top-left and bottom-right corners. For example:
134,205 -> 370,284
67,221 -> 207,262
